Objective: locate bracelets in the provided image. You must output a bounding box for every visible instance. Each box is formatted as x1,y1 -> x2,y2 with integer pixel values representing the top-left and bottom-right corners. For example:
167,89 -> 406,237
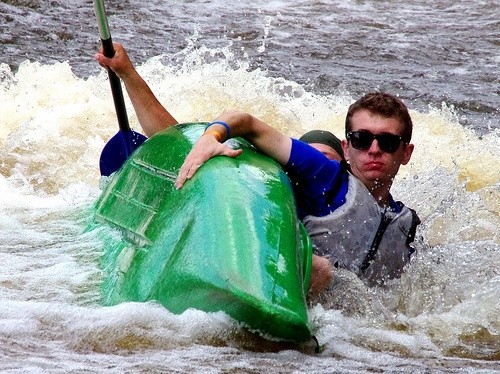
204,122 -> 231,140
200,132 -> 222,141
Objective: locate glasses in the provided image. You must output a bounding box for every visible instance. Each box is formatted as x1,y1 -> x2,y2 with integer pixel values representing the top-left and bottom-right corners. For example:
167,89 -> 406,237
345,130 -> 404,155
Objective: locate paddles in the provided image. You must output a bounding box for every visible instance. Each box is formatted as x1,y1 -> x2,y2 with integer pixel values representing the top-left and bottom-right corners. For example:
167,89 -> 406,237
82,0 -> 153,178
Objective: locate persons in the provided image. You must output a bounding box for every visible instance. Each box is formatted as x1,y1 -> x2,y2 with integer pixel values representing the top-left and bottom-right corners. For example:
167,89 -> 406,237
170,90 -> 421,310
97,41 -> 347,164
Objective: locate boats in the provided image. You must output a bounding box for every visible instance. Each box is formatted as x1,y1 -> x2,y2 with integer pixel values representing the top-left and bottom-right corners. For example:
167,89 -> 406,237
80,121 -> 313,347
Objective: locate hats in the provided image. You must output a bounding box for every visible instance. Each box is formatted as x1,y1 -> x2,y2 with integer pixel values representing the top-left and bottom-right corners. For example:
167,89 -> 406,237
299,131 -> 347,162
